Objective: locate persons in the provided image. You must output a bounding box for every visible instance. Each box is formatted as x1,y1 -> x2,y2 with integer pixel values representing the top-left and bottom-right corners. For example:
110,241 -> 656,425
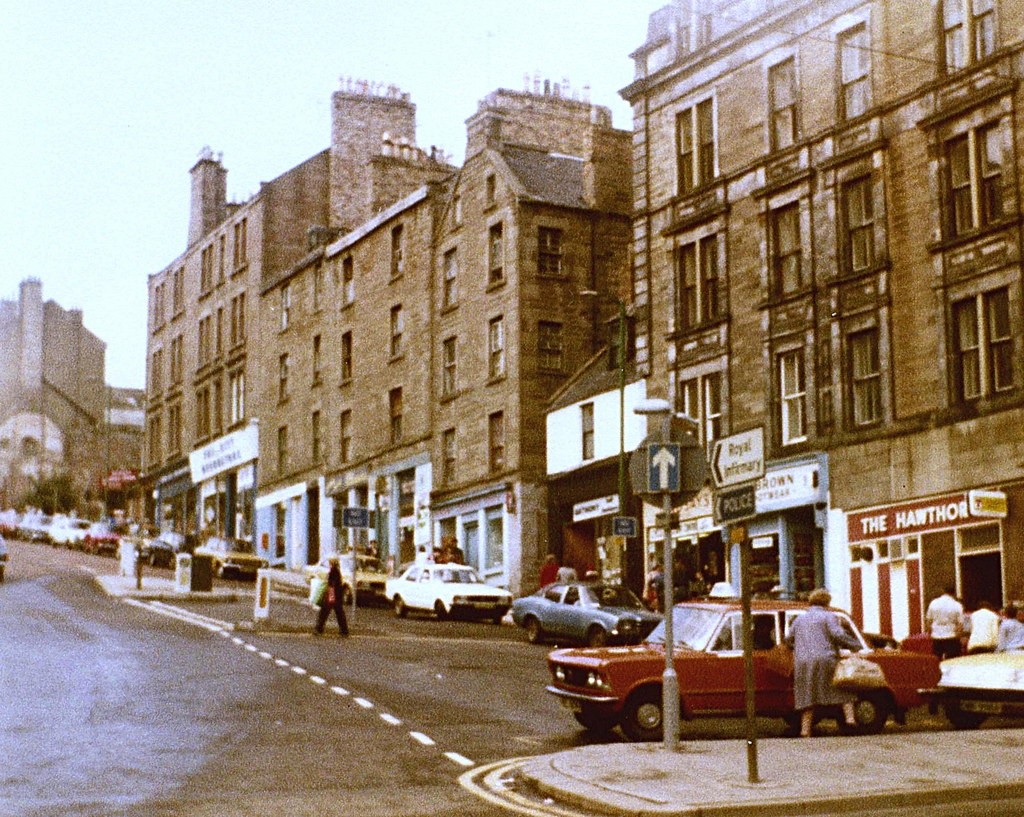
787,590 -> 864,738
314,536 -> 469,638
540,553 -> 713,614
925,581 -> 1024,715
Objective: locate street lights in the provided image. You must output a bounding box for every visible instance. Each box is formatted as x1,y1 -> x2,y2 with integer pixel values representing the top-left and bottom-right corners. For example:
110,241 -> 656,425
579,286 -> 636,604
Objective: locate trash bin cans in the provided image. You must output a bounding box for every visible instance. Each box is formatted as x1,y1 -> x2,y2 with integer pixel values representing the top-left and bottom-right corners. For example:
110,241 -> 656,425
175,551 -> 213,593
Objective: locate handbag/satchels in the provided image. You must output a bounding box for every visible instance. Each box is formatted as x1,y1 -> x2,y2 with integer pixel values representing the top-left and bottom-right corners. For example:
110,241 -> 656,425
832,657 -> 888,690
326,586 -> 336,604
309,578 -> 327,611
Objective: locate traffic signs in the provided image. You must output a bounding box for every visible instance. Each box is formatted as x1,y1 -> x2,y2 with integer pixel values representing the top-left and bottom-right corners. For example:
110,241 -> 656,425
708,424 -> 769,488
611,515 -> 638,538
710,487 -> 756,528
342,508 -> 372,529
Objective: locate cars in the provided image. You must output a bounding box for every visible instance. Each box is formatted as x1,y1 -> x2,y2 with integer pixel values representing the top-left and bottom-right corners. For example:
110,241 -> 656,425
303,552 -> 392,606
511,577 -> 664,647
544,596 -> 947,741
191,535 -> 270,581
0,506 -> 197,578
382,563 -> 512,625
933,647 -> 1024,731
